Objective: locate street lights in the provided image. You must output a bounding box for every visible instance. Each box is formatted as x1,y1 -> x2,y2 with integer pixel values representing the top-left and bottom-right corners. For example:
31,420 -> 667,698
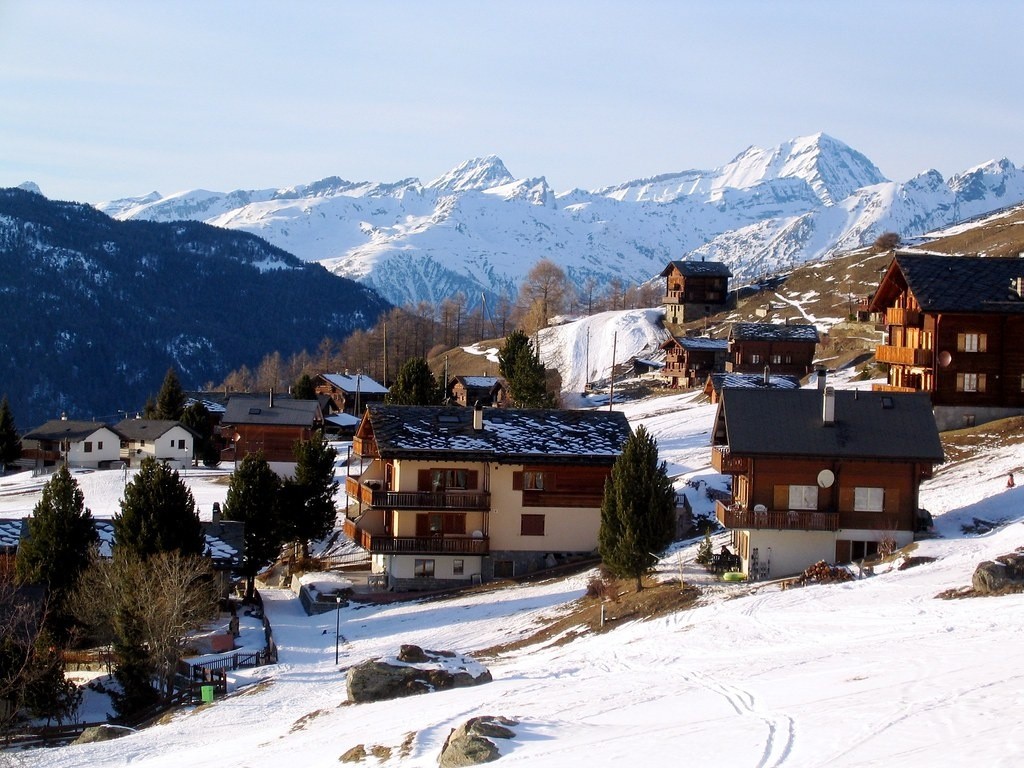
335,596 -> 343,665
185,446 -> 188,477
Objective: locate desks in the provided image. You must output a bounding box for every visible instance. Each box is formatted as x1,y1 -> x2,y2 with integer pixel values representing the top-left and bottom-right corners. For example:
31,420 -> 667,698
367,573 -> 388,588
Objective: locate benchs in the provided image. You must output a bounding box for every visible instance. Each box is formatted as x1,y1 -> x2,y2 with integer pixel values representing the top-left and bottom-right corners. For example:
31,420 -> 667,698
711,554 -> 740,573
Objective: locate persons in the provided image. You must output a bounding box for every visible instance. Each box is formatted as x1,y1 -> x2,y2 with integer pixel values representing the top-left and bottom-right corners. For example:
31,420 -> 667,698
721,545 -> 731,560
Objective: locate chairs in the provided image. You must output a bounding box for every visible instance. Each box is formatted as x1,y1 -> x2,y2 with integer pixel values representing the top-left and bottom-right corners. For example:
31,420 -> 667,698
809,513 -> 825,530
754,504 -> 767,526
787,511 -> 799,528
728,501 -> 740,511
